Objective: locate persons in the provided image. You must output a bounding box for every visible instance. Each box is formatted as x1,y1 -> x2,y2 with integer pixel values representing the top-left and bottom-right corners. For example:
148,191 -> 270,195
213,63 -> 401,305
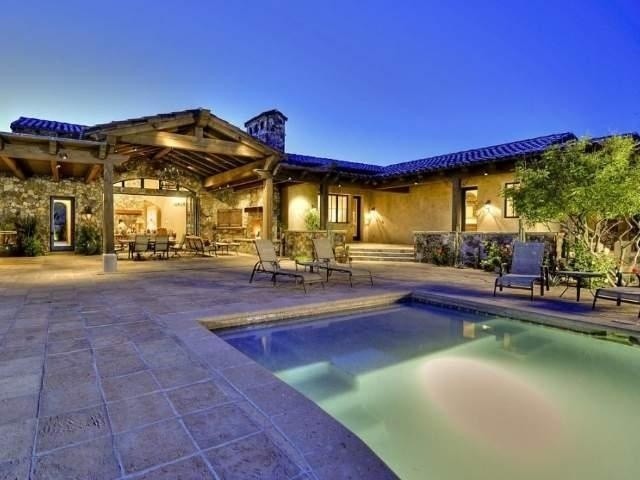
118,219 -> 125,234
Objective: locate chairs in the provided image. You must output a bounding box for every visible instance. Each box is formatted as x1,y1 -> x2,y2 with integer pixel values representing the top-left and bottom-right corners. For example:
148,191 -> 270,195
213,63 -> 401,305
493,240 -> 639,318
113,223 -> 241,259
249,235 -> 375,293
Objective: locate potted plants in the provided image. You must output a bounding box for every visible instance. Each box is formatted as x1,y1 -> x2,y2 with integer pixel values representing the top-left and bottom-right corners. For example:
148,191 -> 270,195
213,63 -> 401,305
428,242 -> 452,265
0,213 -> 51,257
477,241 -> 514,272
75,214 -> 103,256
